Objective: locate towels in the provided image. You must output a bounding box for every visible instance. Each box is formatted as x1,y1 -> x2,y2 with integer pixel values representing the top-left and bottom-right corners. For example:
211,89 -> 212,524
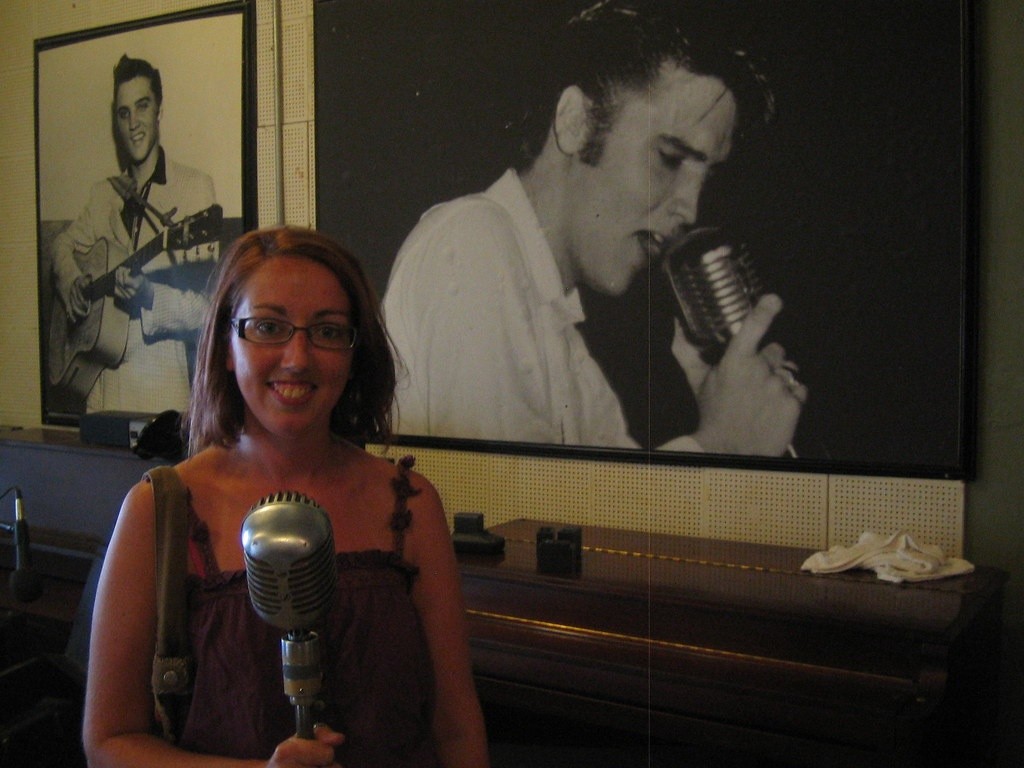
801,529 -> 976,584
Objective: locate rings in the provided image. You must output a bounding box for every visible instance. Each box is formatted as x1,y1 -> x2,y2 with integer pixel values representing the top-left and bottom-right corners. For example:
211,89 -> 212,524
773,365 -> 798,385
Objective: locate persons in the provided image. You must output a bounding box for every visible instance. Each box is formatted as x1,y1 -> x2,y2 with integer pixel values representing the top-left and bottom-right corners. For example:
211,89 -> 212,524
81,224 -> 491,768
378,0 -> 809,461
48,53 -> 220,447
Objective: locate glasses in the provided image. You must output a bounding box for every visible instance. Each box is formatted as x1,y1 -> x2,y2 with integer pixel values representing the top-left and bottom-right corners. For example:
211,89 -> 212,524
230,316 -> 360,350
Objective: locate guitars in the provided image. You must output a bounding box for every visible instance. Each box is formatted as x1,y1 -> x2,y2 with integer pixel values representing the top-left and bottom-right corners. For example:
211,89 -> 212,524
48,204 -> 223,409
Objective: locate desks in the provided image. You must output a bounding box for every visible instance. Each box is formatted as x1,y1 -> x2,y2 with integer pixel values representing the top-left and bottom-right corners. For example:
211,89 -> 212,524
458,518 -> 1011,768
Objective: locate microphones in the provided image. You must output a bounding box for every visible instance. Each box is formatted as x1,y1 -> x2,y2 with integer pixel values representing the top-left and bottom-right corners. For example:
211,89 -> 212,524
13,489 -> 30,572
242,492 -> 339,703
668,228 -> 801,459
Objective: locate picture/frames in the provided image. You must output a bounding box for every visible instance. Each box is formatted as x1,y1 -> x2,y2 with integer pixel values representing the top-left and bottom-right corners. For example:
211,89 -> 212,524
32,0 -> 257,428
312,0 -> 980,480
